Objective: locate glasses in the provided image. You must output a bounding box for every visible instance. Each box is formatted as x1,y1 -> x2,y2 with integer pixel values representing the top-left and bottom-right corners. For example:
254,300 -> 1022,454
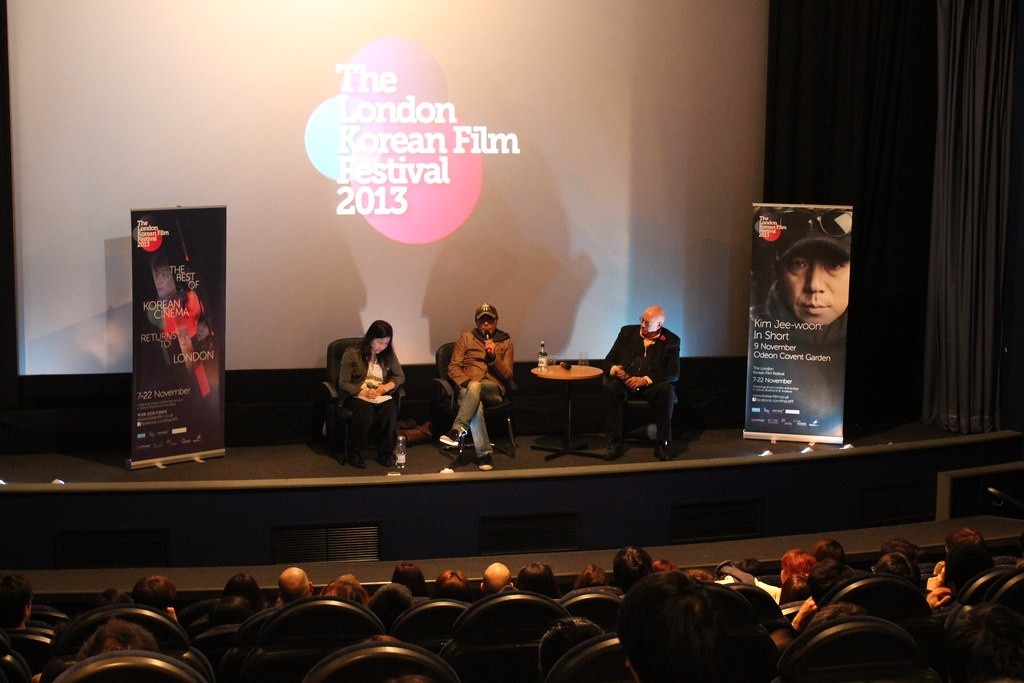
777,211 -> 852,241
639,317 -> 653,327
870,565 -> 881,573
479,317 -> 495,324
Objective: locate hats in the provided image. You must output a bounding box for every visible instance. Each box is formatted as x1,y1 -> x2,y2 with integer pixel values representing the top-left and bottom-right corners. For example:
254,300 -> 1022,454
475,303 -> 499,324
774,209 -> 853,273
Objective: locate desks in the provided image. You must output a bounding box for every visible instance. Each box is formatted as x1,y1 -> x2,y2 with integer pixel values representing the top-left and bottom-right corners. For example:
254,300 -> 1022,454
529,366 -> 606,461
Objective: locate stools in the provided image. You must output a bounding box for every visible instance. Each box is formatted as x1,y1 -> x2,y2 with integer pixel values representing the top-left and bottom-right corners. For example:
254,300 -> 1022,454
619,389 -> 677,445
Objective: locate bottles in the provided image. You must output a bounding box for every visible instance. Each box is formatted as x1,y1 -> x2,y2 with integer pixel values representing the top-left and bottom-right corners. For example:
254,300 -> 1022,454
395,436 -> 407,468
538,340 -> 547,372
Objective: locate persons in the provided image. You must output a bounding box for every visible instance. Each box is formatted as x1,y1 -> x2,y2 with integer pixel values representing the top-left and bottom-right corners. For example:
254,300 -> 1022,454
602,305 -> 681,462
0,526 -> 1023,683
754,207 -> 852,345
438,304 -> 515,469
339,319 -> 405,469
150,252 -> 210,356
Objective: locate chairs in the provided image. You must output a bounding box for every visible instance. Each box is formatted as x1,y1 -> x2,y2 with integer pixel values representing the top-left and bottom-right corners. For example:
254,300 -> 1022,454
432,341 -> 516,461
1,558 -> 1024,682
322,337 -> 406,466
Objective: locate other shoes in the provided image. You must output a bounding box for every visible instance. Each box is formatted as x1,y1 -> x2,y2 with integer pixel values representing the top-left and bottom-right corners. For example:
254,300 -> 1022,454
654,441 -> 671,462
604,436 -> 625,460
347,451 -> 366,467
479,453 -> 494,470
375,453 -> 392,466
440,429 -> 460,446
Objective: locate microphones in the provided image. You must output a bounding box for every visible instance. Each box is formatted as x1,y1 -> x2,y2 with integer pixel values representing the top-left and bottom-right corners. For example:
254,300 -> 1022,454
560,362 -> 571,370
367,382 -> 391,396
485,329 -> 491,356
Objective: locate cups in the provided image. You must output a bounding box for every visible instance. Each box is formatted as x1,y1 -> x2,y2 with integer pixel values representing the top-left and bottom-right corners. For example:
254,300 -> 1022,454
579,351 -> 588,370
546,356 -> 556,371
